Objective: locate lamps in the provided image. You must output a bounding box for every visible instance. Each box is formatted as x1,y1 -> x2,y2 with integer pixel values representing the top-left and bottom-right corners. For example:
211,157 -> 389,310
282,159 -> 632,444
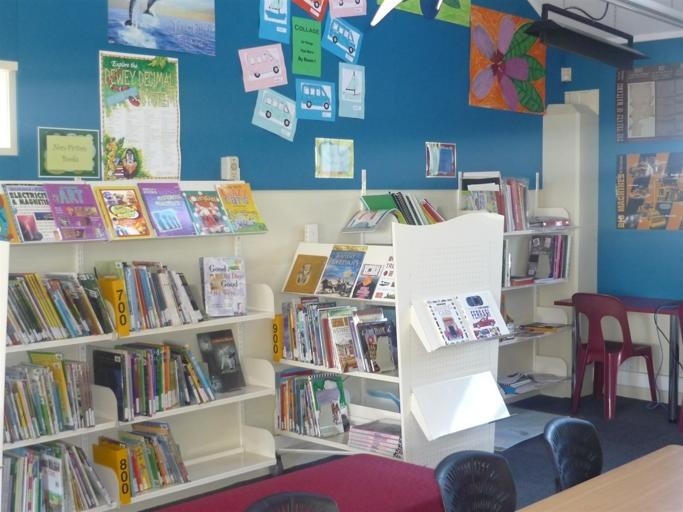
528,4 -> 648,74
599,1 -> 683,31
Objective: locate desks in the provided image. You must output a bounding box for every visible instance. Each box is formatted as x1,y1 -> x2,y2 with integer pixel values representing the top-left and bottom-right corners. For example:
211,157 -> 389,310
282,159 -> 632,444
552,291 -> 680,424
518,442 -> 683,512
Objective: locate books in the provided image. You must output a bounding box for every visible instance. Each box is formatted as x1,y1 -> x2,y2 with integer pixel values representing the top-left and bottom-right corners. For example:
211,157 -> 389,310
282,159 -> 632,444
2,181 -> 264,512
520,322 -> 567,333
273,192 -> 448,459
498,373 -> 564,394
410,291 -> 509,352
461,171 -> 569,286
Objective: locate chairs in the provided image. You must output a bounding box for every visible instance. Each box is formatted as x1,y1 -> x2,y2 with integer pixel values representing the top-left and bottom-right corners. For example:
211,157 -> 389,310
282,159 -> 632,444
544,416 -> 605,494
432,449 -> 519,512
569,291 -> 660,417
244,493 -> 340,512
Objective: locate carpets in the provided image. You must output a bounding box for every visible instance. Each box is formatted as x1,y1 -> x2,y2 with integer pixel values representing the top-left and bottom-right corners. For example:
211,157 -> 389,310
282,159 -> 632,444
146,450 -> 458,512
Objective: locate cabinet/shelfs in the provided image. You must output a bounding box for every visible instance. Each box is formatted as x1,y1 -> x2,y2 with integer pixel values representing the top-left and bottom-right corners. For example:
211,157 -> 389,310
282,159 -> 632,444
2,181 -> 281,512
499,231 -> 578,397
282,210 -> 505,473
